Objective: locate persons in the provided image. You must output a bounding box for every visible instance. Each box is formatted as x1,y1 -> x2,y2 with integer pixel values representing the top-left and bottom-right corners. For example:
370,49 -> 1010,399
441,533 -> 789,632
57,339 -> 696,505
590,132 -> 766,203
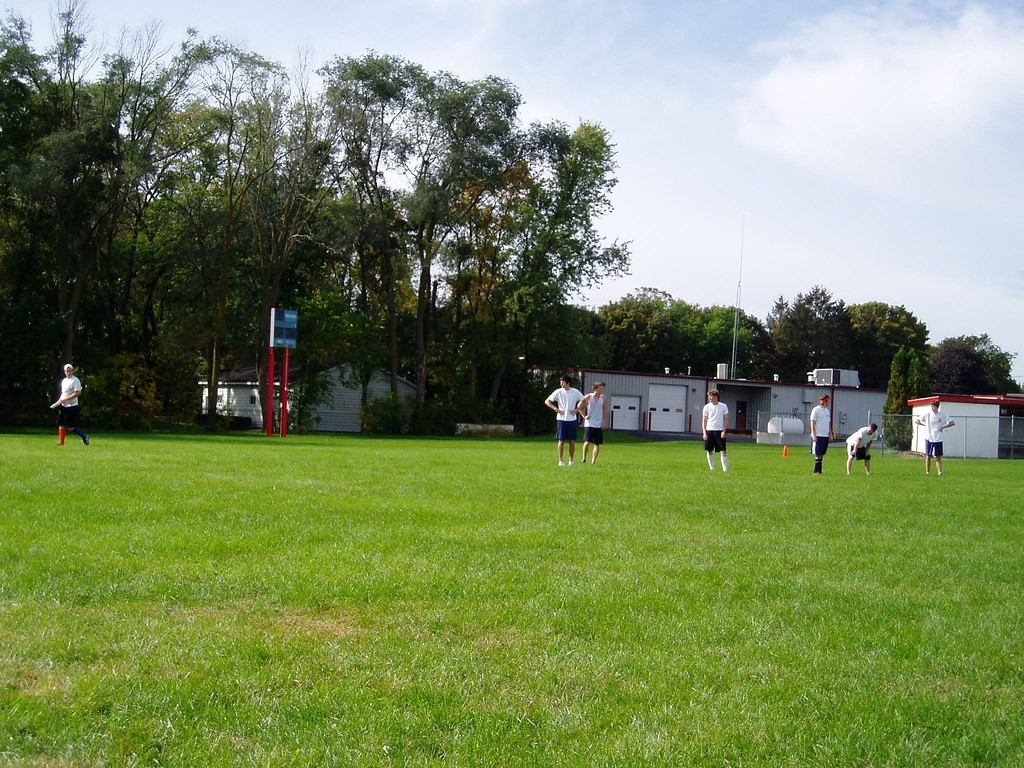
545,374 -> 585,467
915,401 -> 955,475
810,394 -> 834,476
846,423 -> 878,475
577,381 -> 609,464
50,364 -> 89,445
702,389 -> 729,472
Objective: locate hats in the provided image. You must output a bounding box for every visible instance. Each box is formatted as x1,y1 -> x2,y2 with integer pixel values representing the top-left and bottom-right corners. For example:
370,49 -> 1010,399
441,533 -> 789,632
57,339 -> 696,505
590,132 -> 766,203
820,398 -> 830,404
930,404 -> 938,407
64,364 -> 73,370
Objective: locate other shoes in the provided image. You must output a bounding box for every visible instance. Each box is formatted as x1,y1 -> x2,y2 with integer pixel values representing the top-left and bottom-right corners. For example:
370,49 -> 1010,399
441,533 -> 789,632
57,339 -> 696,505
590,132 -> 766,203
84,434 -> 89,445
938,471 -> 943,476
581,459 -> 586,463
814,471 -> 821,475
